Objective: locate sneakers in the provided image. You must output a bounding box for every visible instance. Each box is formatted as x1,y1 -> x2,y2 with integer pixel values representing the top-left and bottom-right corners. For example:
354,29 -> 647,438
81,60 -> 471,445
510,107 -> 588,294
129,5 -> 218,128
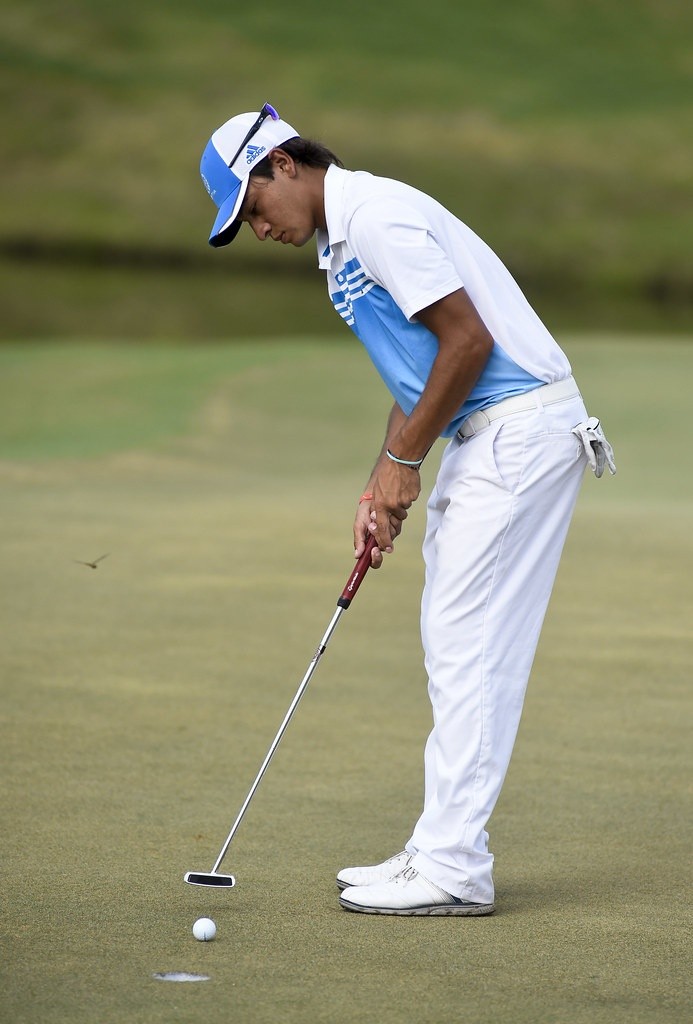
335,850 -> 414,889
338,864 -> 496,916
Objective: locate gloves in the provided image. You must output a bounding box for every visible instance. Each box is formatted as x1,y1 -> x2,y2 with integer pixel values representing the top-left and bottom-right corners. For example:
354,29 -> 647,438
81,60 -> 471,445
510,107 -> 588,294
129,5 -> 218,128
571,417 -> 617,478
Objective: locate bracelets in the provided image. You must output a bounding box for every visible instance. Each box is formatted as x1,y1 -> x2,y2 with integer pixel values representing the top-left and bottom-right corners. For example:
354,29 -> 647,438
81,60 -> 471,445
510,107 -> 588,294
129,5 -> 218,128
359,494 -> 372,505
387,449 -> 423,470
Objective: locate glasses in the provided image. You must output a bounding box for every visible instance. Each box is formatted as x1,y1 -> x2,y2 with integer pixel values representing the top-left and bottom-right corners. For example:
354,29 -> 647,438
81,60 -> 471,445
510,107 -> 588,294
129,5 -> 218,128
228,100 -> 280,171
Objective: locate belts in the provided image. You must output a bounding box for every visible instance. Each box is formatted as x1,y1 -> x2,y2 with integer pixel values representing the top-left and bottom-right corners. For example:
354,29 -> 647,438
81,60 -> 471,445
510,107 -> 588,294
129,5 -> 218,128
456,376 -> 580,438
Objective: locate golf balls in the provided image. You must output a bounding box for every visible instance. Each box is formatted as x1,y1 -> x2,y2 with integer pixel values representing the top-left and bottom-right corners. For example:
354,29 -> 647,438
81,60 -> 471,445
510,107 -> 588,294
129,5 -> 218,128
192,917 -> 217,942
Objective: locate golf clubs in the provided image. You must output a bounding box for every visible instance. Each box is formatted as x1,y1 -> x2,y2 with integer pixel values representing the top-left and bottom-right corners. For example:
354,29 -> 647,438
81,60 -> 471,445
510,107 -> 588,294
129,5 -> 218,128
182,533 -> 378,889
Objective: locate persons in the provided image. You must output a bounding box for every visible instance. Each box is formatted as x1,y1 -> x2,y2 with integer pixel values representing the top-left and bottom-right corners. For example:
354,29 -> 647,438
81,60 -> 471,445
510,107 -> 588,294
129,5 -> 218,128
199,100 -> 616,918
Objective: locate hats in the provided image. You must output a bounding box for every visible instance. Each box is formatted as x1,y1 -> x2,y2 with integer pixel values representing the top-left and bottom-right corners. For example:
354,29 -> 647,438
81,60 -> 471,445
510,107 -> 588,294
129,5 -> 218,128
200,111 -> 300,248
